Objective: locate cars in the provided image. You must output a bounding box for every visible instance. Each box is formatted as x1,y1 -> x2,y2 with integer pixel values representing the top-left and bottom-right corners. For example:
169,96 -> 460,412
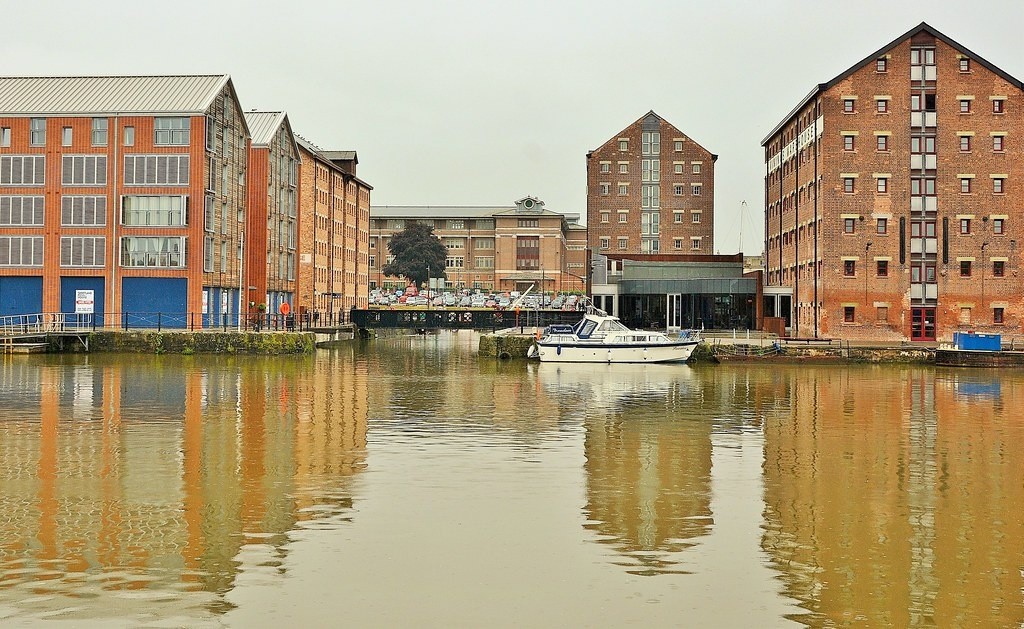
551,294 -> 578,309
485,291 -> 551,308
369,286 -> 435,306
432,288 -> 485,308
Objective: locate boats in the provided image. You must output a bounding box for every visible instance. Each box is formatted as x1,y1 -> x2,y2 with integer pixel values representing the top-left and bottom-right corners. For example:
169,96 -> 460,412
533,285 -> 705,362
934,338 -> 1023,368
415,327 -> 441,335
